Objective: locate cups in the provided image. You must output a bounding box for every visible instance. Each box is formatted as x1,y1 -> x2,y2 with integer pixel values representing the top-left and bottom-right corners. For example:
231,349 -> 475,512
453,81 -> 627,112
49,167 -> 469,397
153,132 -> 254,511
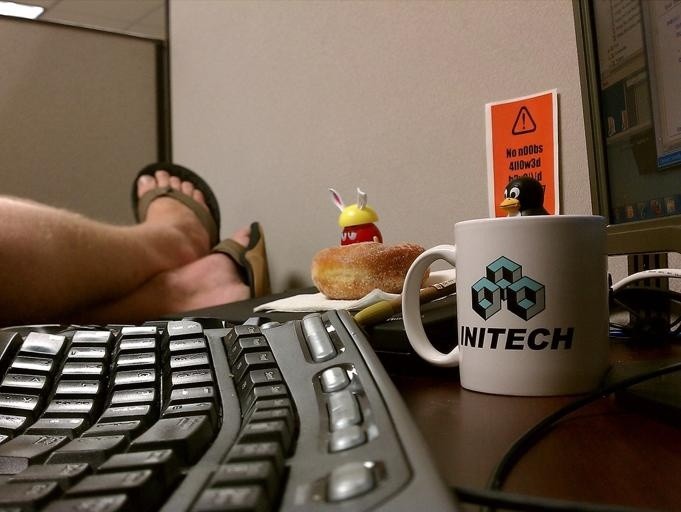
401,216 -> 611,398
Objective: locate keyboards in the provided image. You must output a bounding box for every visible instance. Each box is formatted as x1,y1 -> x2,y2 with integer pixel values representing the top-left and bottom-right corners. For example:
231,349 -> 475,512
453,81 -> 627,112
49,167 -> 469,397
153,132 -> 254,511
0,309 -> 455,512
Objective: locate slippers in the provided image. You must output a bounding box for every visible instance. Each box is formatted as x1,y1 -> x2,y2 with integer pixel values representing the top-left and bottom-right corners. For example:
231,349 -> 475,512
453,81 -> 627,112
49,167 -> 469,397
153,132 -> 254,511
212,221 -> 271,299
132,163 -> 222,251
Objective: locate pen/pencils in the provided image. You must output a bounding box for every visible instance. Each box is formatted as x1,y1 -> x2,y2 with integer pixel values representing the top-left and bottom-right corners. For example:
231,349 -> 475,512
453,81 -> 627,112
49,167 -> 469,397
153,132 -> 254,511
353,277 -> 456,325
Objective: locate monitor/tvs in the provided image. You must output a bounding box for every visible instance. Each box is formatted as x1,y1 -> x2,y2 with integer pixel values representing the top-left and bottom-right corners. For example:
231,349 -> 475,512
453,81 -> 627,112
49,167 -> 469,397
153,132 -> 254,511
572,0 -> 681,256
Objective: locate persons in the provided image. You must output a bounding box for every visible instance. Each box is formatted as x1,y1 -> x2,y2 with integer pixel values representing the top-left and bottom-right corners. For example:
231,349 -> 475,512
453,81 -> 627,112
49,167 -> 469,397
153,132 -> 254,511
0,161 -> 267,325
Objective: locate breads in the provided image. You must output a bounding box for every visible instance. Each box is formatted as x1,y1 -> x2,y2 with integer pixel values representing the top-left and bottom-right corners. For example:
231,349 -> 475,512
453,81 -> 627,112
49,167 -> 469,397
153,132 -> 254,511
312,241 -> 430,300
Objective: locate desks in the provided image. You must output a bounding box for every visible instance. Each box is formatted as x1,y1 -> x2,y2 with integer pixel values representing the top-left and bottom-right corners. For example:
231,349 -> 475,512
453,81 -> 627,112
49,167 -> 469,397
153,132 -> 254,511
387,340 -> 679,512
